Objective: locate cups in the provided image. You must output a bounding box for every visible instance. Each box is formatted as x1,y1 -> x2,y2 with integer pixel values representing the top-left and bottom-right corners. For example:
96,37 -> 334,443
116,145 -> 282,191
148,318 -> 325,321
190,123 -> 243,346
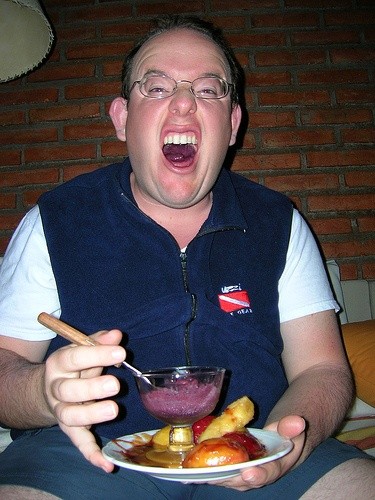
136,365 -> 227,455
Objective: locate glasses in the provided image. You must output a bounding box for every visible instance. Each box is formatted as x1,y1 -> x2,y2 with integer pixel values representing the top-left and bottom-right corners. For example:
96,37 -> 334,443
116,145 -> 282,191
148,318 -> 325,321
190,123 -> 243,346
126,71 -> 234,108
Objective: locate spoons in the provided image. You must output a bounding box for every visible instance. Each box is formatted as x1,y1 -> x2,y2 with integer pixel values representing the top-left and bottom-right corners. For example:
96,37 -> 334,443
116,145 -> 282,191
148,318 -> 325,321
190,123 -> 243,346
37,312 -> 182,397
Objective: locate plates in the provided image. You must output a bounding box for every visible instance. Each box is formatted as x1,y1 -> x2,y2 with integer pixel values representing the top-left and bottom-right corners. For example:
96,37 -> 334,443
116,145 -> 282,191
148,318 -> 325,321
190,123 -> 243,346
102,427 -> 295,481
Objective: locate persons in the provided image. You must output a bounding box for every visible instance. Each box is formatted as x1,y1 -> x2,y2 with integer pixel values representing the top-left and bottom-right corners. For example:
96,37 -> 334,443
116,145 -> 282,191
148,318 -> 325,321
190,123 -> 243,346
0,15 -> 375,500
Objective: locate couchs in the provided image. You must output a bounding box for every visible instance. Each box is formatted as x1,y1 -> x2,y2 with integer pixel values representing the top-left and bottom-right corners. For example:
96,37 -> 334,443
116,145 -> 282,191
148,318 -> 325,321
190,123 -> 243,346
0,280 -> 375,452
